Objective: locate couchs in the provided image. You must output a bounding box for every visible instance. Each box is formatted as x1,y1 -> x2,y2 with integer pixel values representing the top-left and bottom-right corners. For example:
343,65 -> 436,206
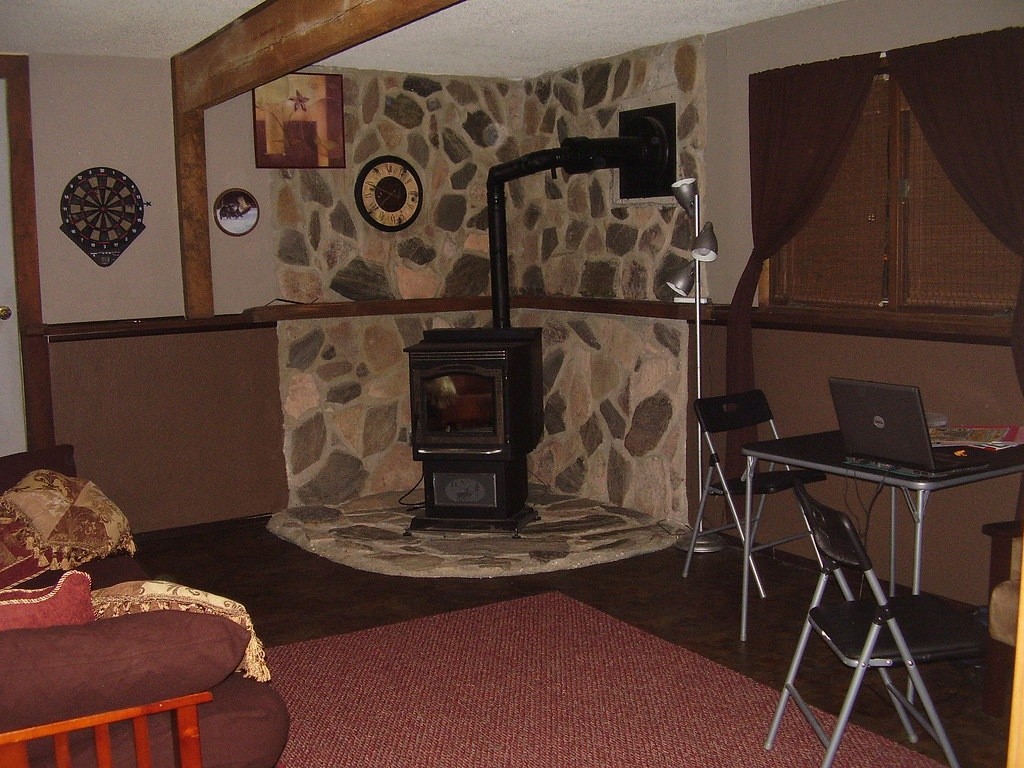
0,444 -> 290,768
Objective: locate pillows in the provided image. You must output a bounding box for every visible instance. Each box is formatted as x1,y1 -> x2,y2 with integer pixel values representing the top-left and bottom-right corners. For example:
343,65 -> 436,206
0,469 -> 270,682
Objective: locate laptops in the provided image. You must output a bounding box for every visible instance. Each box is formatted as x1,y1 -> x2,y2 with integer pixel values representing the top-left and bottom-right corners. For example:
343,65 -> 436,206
829,375 -> 997,479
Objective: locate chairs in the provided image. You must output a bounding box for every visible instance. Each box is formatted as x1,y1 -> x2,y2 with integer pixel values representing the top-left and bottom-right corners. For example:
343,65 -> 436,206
764,483 -> 997,768
983,471 -> 1024,718
682,389 -> 829,597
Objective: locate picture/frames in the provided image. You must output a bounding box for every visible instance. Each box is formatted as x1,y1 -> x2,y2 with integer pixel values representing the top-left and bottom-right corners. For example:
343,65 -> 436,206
213,188 -> 260,237
252,72 -> 346,169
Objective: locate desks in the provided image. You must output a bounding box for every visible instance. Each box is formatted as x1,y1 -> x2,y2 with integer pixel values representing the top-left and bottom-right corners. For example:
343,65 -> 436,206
740,430 -> 1024,641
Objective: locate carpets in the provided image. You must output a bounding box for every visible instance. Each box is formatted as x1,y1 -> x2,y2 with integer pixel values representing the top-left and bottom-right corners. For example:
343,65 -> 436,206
246,590 -> 948,768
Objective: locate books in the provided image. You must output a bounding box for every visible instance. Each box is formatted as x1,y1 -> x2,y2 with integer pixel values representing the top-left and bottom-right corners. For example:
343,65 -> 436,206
929,423 -> 1024,453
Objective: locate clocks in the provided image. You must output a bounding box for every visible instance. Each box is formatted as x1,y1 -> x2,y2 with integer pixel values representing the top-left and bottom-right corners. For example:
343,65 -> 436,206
354,155 -> 423,232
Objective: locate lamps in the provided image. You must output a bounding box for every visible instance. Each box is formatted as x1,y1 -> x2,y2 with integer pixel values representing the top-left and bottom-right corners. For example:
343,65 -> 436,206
665,178 -> 726,553
